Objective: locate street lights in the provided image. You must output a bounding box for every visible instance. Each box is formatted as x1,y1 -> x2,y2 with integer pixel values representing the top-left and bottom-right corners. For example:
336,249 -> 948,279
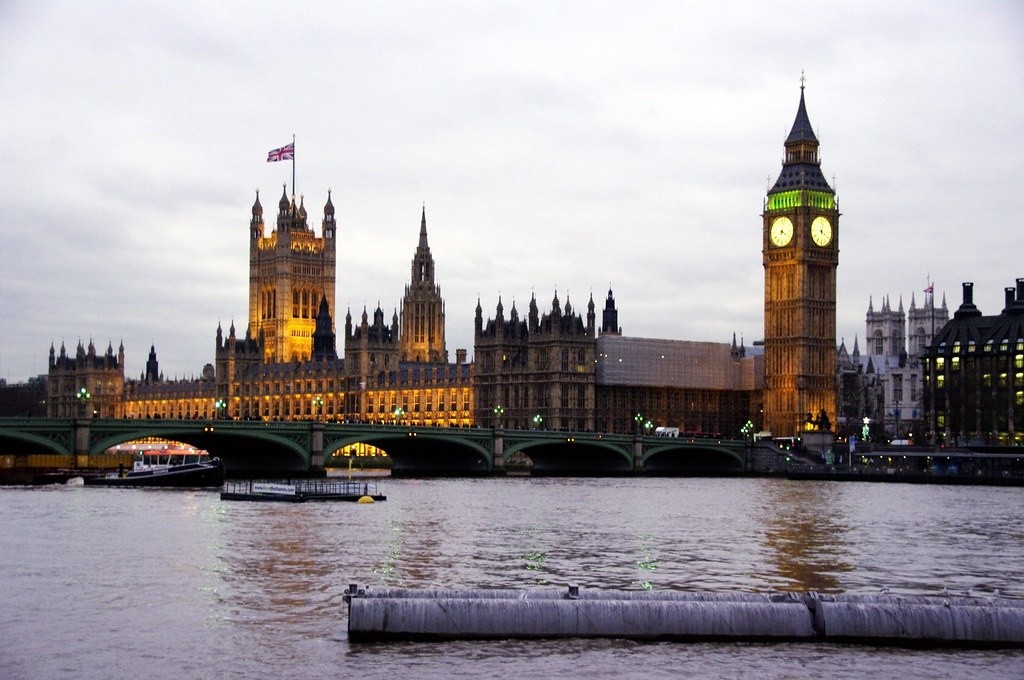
78,387 -> 90,418
494,406 -> 504,428
216,399 -> 226,419
746,420 -> 753,437
535,415 -> 542,430
396,407 -> 404,425
646,420 -> 653,434
313,396 -> 323,422
635,413 -> 643,433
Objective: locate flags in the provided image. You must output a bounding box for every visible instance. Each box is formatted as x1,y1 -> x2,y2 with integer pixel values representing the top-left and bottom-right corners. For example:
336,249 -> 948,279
267,143 -> 294,163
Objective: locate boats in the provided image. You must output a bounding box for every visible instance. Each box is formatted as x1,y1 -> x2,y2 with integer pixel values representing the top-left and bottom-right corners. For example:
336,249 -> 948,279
20,457 -> 224,489
